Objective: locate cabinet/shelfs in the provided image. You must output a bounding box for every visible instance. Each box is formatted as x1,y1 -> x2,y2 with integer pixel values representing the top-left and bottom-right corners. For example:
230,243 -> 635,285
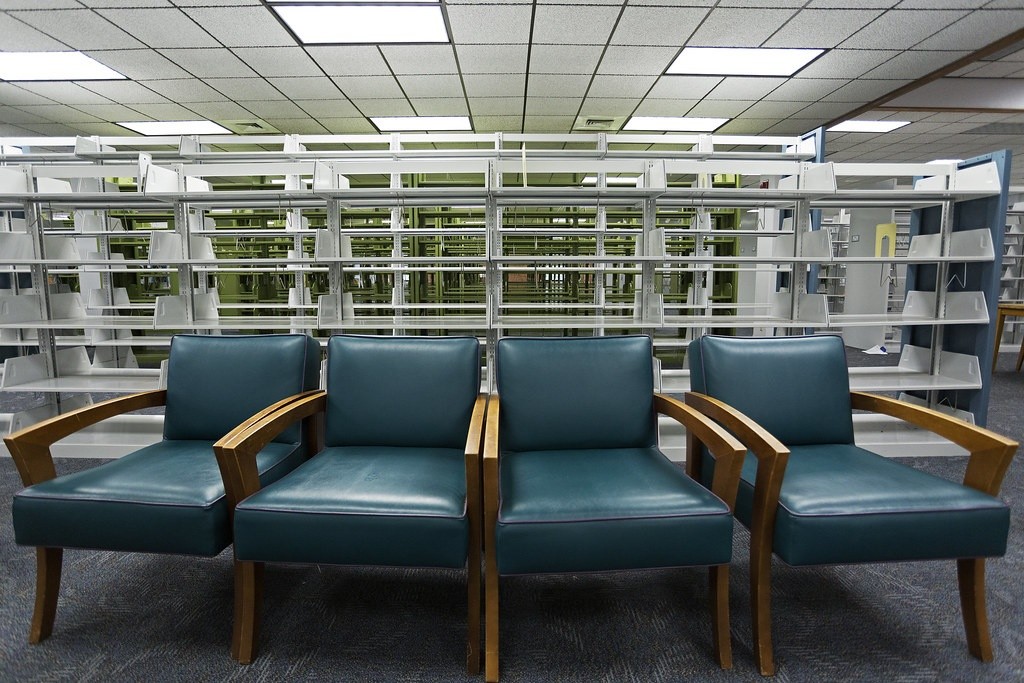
0,127 -> 1024,457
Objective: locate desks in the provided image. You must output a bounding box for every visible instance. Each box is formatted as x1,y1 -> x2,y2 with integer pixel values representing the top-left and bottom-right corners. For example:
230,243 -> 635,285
991,304 -> 1024,373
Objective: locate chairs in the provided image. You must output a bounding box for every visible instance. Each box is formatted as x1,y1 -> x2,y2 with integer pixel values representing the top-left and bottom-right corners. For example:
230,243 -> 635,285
4,333 -> 321,661
482,334 -> 748,683
221,334 -> 489,675
686,335 -> 1020,676
